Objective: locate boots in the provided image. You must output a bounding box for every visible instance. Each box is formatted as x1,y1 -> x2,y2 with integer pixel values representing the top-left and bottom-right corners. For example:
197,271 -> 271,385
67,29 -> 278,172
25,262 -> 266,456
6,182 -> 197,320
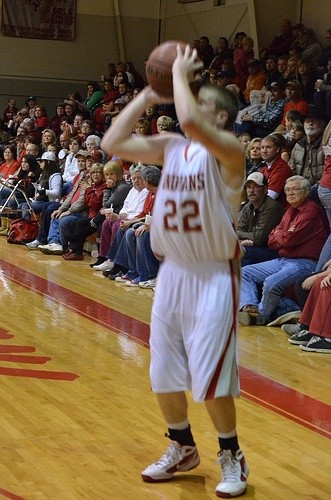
0,217 -> 11,236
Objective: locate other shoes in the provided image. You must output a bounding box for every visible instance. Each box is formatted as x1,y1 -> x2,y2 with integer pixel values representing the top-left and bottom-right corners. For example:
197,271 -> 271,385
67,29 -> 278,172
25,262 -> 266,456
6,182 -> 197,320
62,251 -> 84,260
237,305 -> 266,326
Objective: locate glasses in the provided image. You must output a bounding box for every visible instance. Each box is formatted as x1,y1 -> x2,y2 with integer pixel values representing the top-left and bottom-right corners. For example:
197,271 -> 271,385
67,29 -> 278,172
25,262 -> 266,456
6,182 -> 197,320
304,120 -> 324,124
284,188 -> 309,194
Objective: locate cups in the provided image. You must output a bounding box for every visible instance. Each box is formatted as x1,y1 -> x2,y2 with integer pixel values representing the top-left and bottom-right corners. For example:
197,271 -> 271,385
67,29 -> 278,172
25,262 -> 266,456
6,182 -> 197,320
145,214 -> 152,225
104,208 -> 114,219
317,80 -> 324,92
9,175 -> 14,184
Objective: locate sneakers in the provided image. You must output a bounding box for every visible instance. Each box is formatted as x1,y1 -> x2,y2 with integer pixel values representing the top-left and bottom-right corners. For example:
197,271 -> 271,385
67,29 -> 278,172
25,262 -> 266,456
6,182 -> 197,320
38,243 -> 63,255
215,449 -> 250,498
288,330 -> 313,345
26,239 -> 43,250
89,256 -> 156,292
141,433 -> 200,483
300,336 -> 331,353
281,324 -> 301,336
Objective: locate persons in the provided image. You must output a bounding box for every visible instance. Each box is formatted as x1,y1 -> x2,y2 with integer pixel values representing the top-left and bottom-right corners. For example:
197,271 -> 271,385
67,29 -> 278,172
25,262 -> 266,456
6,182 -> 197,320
101,43 -> 250,496
0,19 -> 331,353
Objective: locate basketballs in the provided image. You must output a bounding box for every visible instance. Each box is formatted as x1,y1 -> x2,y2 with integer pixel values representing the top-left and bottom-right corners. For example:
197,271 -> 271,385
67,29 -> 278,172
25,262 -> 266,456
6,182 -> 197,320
145,40 -> 200,100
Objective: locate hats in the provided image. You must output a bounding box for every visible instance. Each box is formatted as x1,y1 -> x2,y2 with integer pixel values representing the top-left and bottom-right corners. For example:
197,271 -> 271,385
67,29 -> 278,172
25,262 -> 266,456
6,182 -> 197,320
266,82 -> 280,91
213,71 -> 228,79
75,149 -> 86,159
36,151 -> 56,162
115,98 -> 127,106
86,150 -> 102,163
299,106 -> 329,125
19,109 -> 29,115
284,79 -> 301,88
244,172 -> 267,189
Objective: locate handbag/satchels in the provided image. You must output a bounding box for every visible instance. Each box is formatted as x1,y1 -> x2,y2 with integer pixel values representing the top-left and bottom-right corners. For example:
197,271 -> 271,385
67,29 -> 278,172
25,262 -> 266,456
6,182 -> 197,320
7,218 -> 39,245
